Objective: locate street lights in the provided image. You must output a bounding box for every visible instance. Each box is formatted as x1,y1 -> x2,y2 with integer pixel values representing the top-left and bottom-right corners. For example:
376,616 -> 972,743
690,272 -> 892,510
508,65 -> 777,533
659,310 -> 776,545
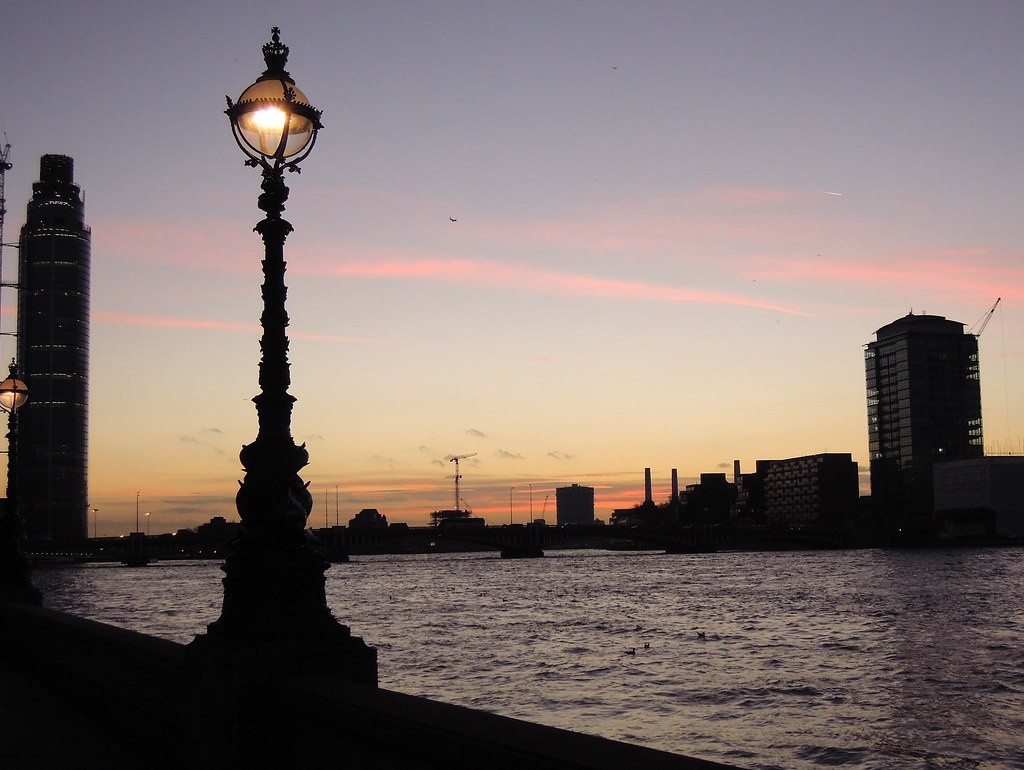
136,492 -> 139,533
92,509 -> 98,538
179,24 -> 378,717
0,358 -> 37,604
145,513 -> 151,536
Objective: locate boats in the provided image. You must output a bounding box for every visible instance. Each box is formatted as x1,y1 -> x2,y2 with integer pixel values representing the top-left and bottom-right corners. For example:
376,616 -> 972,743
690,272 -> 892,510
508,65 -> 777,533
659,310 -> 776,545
500,544 -> 544,559
118,538 -> 151,568
324,550 -> 349,563
